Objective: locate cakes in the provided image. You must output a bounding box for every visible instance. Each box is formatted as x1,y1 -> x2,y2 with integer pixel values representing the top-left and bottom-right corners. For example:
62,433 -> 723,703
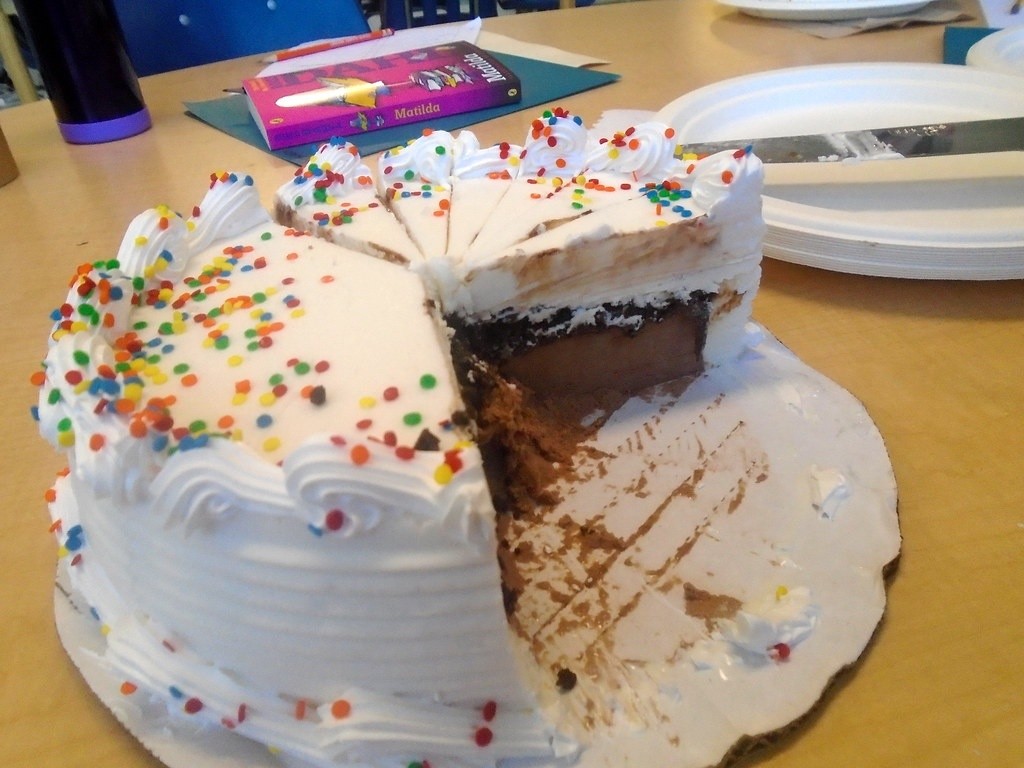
28,102 -> 766,768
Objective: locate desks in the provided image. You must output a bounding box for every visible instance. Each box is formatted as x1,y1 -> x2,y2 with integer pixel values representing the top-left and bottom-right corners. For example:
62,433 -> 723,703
0,1 -> 1024,768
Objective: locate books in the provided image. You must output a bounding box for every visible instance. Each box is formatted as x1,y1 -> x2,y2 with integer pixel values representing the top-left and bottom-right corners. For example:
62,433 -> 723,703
239,39 -> 524,153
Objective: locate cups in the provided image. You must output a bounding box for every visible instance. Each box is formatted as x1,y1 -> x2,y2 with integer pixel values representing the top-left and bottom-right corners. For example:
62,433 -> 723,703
11,0 -> 153,144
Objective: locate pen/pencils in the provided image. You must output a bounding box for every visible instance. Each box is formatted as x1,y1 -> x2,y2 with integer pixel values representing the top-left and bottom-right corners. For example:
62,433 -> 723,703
255,26 -> 395,65
221,87 -> 245,95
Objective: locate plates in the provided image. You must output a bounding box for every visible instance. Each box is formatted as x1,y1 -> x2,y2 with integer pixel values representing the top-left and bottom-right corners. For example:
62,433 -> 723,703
718,0 -> 932,21
652,60 -> 1024,284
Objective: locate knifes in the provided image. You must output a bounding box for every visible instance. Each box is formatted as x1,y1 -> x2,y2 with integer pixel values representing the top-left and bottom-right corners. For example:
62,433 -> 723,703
674,116 -> 1024,163
